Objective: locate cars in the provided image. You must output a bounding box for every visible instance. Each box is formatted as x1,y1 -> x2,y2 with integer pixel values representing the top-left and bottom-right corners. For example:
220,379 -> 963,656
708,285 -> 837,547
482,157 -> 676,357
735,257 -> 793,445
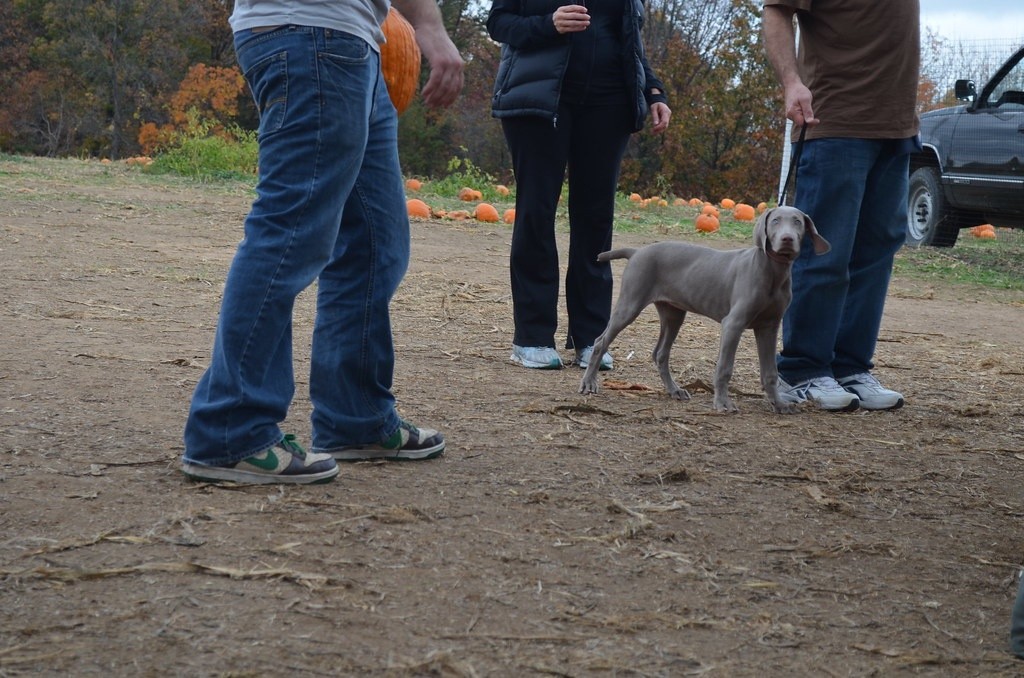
906,44 -> 1024,247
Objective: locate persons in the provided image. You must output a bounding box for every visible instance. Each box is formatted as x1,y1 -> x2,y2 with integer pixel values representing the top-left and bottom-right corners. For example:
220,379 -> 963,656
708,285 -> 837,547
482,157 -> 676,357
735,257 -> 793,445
762,0 -> 920,413
177,2 -> 465,485
487,0 -> 672,370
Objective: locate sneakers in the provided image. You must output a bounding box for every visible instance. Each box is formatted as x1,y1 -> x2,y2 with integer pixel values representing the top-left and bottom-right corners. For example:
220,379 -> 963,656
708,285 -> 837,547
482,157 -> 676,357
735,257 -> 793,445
178,433 -> 340,483
776,372 -> 904,412
310,417 -> 445,460
510,344 -> 563,369
573,346 -> 614,369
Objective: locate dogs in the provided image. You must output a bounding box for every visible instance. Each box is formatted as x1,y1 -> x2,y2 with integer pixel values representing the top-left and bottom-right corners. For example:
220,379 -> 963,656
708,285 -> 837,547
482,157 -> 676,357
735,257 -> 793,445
579,206 -> 831,414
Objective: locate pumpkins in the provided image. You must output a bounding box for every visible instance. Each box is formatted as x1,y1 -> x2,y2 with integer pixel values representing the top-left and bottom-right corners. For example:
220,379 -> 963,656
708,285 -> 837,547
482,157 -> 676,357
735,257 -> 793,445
378,6 -> 420,116
631,193 -> 768,233
404,178 -> 515,224
969,224 -> 996,238
